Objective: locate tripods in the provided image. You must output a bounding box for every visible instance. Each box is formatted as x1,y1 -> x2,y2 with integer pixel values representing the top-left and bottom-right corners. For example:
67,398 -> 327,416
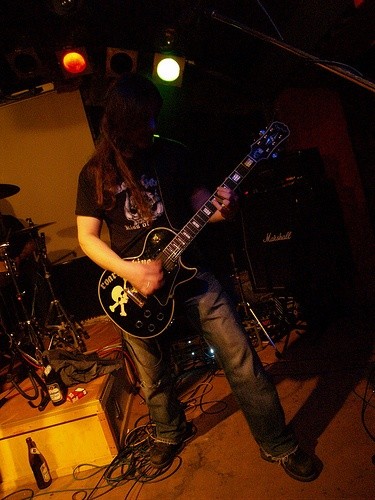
230,254 -> 283,359
2,231 -> 90,367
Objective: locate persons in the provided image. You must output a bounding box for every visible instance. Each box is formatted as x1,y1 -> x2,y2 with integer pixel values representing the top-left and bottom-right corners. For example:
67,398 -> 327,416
75,74 -> 317,483
0,215 -> 35,351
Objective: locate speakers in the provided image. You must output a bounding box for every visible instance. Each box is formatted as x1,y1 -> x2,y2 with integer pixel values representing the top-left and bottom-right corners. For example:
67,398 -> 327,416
240,178 -> 352,294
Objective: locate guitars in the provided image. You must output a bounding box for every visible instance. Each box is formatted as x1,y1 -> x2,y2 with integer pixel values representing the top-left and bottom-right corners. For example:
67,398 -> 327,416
97,118 -> 292,342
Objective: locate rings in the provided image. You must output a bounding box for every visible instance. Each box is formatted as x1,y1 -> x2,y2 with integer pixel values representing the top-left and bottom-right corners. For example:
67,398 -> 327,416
144,281 -> 150,289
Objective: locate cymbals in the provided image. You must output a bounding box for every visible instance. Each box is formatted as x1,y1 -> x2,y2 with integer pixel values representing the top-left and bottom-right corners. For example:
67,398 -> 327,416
17,220 -> 56,234
0,183 -> 21,200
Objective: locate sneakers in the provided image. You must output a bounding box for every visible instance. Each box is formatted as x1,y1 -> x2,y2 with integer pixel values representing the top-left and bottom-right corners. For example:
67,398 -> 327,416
258,443 -> 318,482
149,439 -> 177,468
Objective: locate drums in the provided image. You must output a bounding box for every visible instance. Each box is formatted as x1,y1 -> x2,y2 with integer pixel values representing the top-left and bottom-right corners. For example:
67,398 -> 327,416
0,241 -> 19,277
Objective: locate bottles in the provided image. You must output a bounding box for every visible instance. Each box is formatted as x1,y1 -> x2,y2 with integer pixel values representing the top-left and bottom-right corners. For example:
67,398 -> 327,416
41,354 -> 65,407
25,435 -> 52,488
